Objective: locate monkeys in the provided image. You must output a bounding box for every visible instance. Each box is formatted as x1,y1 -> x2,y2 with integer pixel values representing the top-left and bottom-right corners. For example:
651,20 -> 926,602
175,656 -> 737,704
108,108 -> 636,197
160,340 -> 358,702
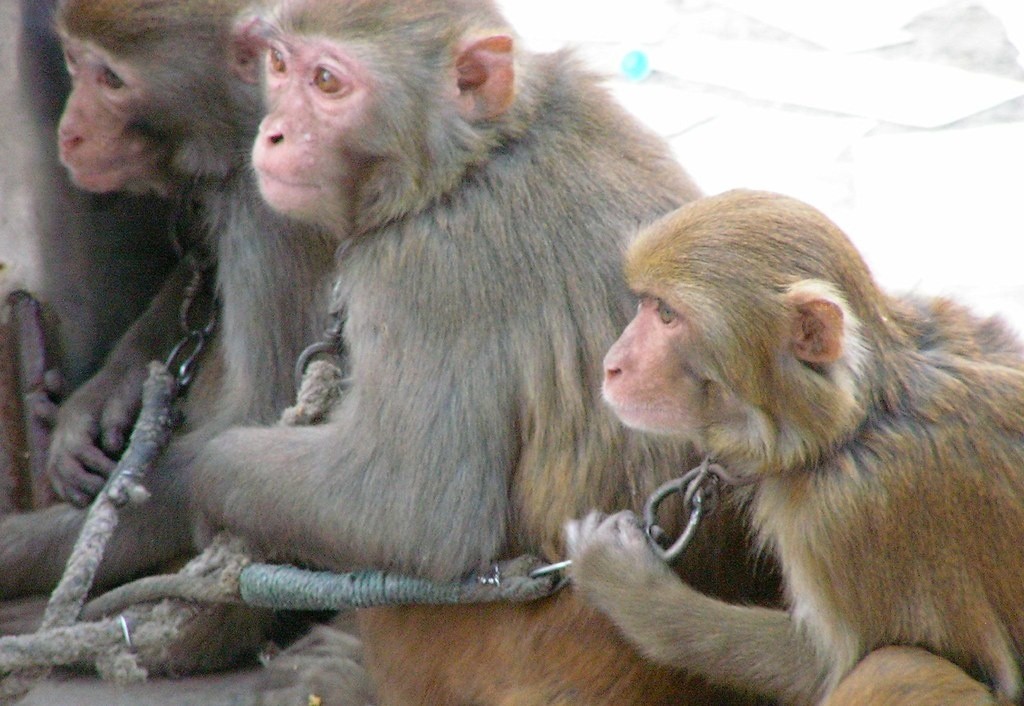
184,1 -> 791,706
558,187 -> 1024,705
0,1 -> 353,678
